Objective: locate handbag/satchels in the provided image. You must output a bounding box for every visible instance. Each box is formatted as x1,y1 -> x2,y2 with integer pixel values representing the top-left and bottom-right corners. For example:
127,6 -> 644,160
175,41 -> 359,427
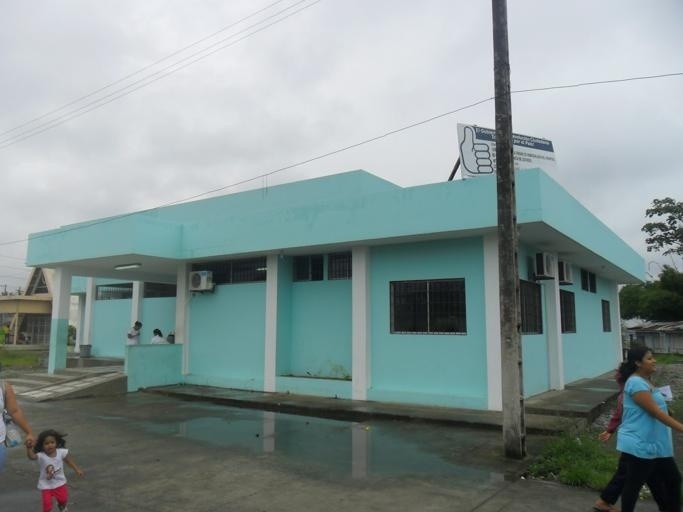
3,414 -> 21,448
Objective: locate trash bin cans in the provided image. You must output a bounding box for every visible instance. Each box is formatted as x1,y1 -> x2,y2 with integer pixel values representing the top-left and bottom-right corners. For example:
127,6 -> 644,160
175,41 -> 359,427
81,346 -> 92,357
5,333 -> 14,345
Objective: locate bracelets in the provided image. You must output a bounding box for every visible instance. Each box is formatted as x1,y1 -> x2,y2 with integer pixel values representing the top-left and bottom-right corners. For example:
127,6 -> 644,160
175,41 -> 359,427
26,446 -> 31,449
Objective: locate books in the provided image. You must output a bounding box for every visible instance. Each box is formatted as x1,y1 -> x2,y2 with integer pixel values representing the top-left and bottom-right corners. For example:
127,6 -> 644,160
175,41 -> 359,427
656,385 -> 673,403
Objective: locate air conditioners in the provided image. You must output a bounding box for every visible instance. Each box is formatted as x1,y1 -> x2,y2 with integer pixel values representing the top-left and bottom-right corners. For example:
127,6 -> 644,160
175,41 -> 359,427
558,261 -> 573,285
189,271 -> 213,291
532,252 -> 556,280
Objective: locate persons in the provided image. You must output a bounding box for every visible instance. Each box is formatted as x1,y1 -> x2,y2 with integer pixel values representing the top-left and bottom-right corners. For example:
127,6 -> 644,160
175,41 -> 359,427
149,329 -> 165,345
615,344 -> 682,512
23,429 -> 83,512
126,320 -> 142,346
0,380 -> 34,476
592,354 -> 673,512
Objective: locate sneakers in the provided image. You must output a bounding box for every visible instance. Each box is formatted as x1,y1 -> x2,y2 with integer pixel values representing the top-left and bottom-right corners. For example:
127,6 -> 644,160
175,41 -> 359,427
592,497 -> 620,512
56,505 -> 67,512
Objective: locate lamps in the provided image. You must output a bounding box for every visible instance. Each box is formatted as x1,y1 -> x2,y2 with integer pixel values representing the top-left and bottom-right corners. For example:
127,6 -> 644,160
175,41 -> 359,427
116,264 -> 142,271
